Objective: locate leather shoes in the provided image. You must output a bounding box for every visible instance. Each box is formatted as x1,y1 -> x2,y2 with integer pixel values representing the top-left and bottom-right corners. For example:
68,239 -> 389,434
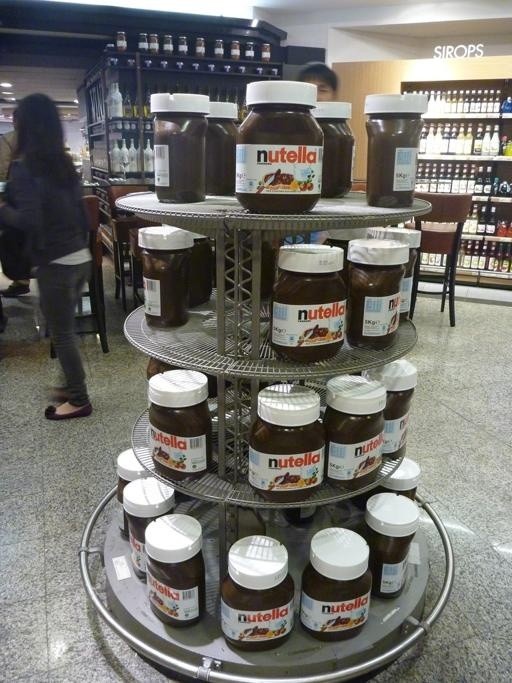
45,403 -> 92,419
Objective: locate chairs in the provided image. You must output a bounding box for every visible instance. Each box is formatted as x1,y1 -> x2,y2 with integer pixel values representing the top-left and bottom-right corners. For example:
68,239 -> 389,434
44,185 -> 161,360
407,189 -> 472,328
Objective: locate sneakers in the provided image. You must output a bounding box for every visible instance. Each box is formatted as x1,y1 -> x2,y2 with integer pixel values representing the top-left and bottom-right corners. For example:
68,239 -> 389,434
0,285 -> 29,298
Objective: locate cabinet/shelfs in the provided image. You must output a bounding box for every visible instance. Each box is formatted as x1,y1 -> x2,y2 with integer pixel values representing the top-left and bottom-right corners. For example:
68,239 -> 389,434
396,78 -> 512,285
79,42 -> 282,267
78,185 -> 456,682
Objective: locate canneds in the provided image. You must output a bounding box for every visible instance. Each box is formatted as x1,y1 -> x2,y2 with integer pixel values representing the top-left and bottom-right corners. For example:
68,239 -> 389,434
301,527 -> 370,641
364,360 -> 418,460
246,384 -> 327,501
233,80 -> 326,210
220,534 -> 296,650
134,223 -> 421,362
377,457 -> 422,501
116,447 -> 209,629
145,371 -> 215,476
150,93 -> 428,212
328,375 -> 386,486
365,492 -> 419,600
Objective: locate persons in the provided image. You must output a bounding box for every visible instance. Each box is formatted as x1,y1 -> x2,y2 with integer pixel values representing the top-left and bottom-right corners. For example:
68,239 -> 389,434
0,91 -> 98,419
295,62 -> 344,101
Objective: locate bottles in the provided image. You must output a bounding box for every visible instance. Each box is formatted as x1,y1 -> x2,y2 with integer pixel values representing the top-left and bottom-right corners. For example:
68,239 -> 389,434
400,85 -> 512,276
109,82 -> 250,177
